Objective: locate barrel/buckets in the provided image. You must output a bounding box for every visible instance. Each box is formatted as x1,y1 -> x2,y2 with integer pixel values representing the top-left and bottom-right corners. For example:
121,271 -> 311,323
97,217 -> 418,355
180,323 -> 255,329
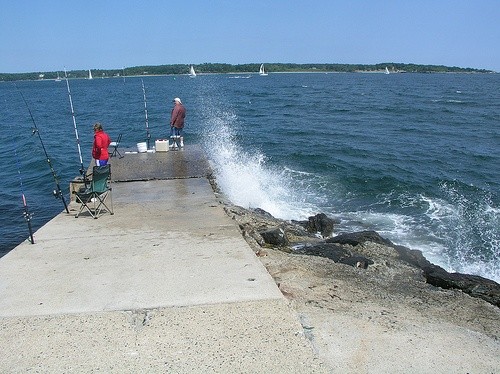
137,142 -> 147,153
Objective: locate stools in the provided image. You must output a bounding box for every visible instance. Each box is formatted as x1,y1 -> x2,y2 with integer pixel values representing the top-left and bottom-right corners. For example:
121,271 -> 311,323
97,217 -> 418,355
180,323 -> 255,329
170,135 -> 182,151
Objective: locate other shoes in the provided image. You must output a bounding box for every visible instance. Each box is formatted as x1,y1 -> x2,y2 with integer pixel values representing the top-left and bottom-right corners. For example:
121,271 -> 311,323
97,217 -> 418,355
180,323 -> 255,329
180,142 -> 184,147
169,143 -> 178,148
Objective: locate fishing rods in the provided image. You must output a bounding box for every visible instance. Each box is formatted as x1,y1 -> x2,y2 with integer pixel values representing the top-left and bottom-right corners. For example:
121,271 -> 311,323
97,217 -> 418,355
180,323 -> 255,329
13,82 -> 69,214
13,145 -> 35,244
141,78 -> 151,149
63,66 -> 87,190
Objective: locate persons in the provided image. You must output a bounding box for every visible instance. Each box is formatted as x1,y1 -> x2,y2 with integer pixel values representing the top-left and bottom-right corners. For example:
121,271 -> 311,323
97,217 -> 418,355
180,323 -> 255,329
92,123 -> 110,166
169,98 -> 185,149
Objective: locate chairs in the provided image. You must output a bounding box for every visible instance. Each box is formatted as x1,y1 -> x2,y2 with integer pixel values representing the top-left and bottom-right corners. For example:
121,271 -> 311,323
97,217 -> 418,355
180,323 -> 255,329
108,133 -> 123,160
71,164 -> 116,219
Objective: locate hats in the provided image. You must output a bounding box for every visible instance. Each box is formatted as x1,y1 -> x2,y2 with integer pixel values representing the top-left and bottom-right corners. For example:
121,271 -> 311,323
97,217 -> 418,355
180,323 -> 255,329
174,97 -> 183,105
